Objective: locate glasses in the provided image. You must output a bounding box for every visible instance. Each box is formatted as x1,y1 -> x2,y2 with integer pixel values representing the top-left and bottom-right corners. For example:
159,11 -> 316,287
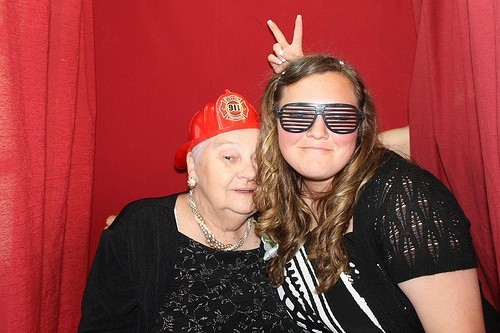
273,103 -> 364,134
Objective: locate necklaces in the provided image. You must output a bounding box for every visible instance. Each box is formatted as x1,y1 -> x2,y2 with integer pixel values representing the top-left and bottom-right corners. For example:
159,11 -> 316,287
188,189 -> 252,250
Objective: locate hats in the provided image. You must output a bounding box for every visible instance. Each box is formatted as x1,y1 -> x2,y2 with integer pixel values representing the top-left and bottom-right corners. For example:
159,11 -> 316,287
173,89 -> 262,170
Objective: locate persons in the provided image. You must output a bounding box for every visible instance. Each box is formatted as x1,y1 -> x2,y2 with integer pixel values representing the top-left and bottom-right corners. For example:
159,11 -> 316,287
104,54 -> 499,332
78,89 -> 290,333
267,13 -> 409,160
